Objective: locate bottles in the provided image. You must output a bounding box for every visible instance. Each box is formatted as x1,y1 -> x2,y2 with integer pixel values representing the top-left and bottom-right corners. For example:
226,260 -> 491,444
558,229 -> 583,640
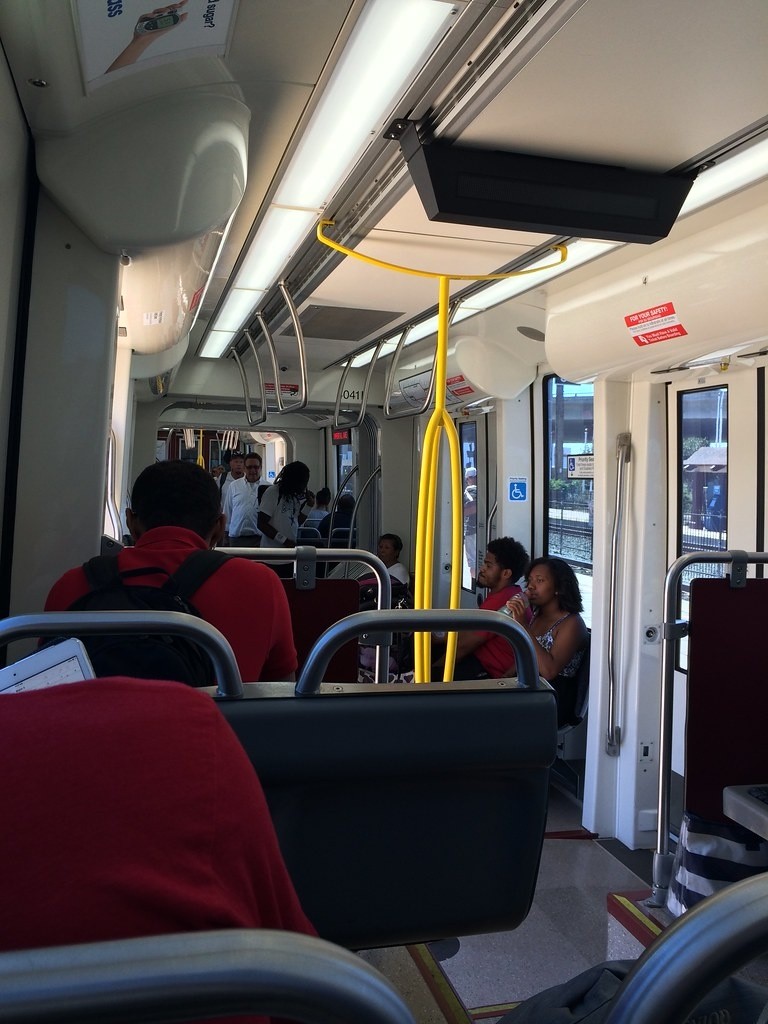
496,590 -> 530,618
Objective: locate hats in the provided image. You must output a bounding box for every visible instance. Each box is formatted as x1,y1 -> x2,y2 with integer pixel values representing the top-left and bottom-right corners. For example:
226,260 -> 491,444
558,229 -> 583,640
231,450 -> 245,458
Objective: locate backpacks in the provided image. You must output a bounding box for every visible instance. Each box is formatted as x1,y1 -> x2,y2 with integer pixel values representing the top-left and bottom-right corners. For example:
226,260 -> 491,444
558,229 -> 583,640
41,543 -> 237,688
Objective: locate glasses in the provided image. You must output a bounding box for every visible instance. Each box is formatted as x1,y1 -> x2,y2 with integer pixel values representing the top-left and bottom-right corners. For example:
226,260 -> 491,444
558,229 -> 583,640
244,464 -> 261,469
218,467 -> 224,470
212,470 -> 217,473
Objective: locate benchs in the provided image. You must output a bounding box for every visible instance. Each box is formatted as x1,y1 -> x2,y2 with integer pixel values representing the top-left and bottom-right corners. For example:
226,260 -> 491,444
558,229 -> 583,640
0,519 -> 768,1024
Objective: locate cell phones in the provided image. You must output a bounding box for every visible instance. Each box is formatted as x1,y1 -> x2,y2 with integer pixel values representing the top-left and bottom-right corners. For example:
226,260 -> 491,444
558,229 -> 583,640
0,637 -> 99,694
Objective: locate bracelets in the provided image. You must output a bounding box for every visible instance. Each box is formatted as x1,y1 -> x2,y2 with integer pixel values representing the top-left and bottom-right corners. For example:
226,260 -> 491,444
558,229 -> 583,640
272,531 -> 288,544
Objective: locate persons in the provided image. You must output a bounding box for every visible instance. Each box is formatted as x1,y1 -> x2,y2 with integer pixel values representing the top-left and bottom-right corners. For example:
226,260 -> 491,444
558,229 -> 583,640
37,458 -> 297,685
404,535 -> 531,682
378,534 -> 411,590
215,463 -> 226,477
257,460 -> 310,578
222,453 -> 274,547
304,487 -> 332,529
317,494 -> 355,537
214,452 -> 245,546
500,557 -> 589,728
